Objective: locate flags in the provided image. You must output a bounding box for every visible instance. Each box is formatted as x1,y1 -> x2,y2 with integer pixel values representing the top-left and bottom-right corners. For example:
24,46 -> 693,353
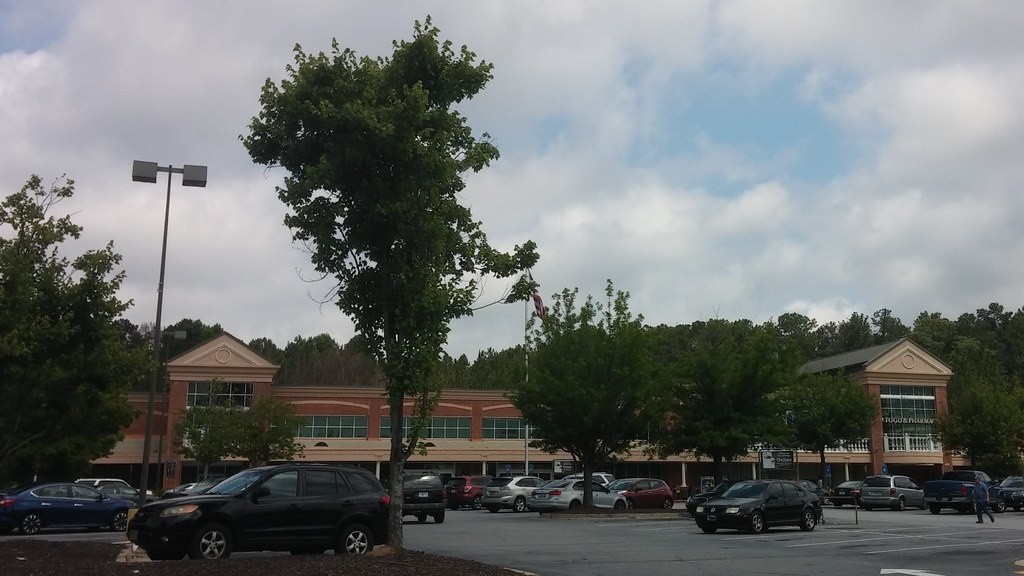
528,268 -> 547,321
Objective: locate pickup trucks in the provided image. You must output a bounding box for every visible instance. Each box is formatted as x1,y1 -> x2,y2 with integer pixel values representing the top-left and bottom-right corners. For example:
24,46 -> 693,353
924,470 -> 1006,515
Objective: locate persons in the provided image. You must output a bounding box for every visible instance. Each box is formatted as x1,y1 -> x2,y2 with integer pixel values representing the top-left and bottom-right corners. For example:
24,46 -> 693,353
970,475 -> 995,524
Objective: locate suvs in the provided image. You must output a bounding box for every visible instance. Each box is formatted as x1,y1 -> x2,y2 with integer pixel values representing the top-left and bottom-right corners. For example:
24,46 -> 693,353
125,463 -> 395,562
398,473 -> 445,525
444,475 -> 489,509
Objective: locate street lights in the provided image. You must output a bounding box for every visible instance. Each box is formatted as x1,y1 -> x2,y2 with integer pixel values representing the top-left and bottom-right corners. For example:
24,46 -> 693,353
132,159 -> 211,504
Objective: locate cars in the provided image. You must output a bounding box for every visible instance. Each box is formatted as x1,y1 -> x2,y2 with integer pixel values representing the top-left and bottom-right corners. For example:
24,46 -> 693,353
75,477 -> 225,504
480,475 -> 549,512
999,476 -> 1024,511
695,480 -> 822,533
563,473 -> 615,487
530,479 -> 629,514
607,477 -> 674,511
686,480 -> 743,519
0,482 -> 134,535
793,479 -> 831,505
860,476 -> 927,511
830,481 -> 863,506
